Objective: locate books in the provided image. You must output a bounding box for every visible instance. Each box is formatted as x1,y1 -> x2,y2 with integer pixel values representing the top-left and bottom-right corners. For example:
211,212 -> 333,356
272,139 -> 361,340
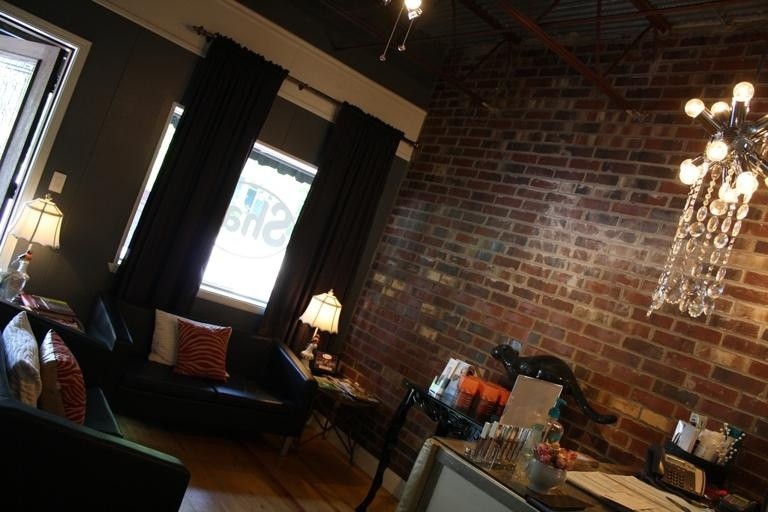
21,293 -> 78,329
523,492 -> 596,512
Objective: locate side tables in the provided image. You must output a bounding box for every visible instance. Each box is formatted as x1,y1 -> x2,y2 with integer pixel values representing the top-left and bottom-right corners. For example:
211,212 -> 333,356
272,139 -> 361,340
0,292 -> 94,341
295,371 -> 385,466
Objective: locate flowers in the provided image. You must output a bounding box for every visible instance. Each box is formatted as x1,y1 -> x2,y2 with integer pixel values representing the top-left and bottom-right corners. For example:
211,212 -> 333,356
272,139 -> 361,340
531,438 -> 578,470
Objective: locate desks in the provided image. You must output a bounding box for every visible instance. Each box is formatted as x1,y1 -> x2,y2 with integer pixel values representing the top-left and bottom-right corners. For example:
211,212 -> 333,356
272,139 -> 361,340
396,436 -> 733,512
353,377 -> 485,512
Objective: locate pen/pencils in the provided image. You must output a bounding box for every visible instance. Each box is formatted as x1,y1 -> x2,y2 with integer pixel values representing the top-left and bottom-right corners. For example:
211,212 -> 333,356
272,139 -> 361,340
665,496 -> 692,512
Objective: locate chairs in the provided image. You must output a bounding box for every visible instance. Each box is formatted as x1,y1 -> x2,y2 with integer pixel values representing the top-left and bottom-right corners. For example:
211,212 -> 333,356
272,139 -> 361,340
0,299 -> 191,512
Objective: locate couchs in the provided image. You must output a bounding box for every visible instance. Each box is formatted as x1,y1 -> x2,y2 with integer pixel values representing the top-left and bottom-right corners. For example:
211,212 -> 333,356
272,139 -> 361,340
89,293 -> 319,447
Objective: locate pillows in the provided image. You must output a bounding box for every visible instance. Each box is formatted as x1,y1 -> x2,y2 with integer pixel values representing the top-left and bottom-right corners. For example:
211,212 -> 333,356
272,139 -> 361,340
2,310 -> 86,424
147,307 -> 233,385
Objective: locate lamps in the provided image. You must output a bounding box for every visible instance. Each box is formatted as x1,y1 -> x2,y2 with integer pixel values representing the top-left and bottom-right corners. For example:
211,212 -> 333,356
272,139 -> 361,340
297,287 -> 342,373
10,193 -> 64,280
378,0 -> 423,61
644,81 -> 768,319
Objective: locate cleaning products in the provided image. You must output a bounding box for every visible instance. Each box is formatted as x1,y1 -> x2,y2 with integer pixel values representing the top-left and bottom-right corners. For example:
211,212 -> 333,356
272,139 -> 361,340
519,395 -> 568,468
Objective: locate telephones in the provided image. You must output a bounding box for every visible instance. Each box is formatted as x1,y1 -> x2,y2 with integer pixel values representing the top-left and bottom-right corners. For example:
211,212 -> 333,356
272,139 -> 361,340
646,446 -> 706,501
